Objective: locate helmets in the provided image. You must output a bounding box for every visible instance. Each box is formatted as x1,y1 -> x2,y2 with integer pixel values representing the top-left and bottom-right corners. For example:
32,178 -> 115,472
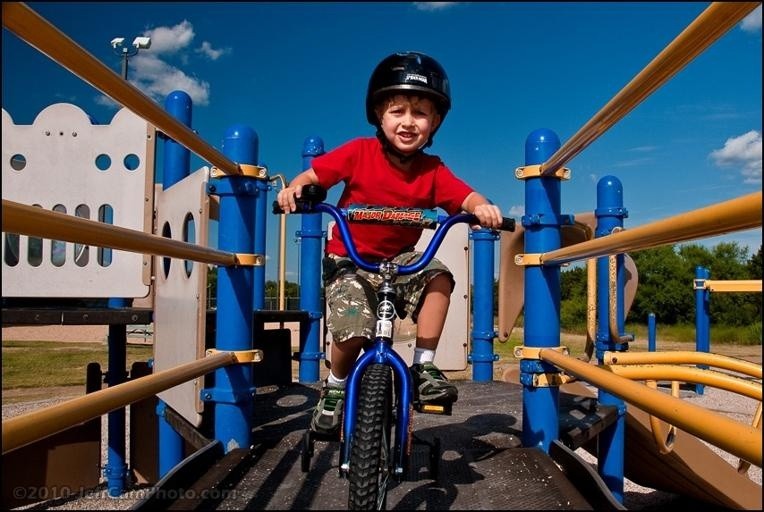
366,51 -> 451,125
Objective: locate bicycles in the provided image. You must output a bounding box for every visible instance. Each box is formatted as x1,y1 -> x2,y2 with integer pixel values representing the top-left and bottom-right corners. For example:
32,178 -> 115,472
272,182 -> 516,510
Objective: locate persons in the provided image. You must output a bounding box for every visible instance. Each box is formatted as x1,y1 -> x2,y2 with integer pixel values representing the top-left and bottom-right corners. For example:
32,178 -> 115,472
278,50 -> 504,435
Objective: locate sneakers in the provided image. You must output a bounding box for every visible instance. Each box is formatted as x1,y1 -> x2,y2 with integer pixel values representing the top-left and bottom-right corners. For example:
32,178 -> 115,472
309,376 -> 346,434
409,360 -> 459,401
